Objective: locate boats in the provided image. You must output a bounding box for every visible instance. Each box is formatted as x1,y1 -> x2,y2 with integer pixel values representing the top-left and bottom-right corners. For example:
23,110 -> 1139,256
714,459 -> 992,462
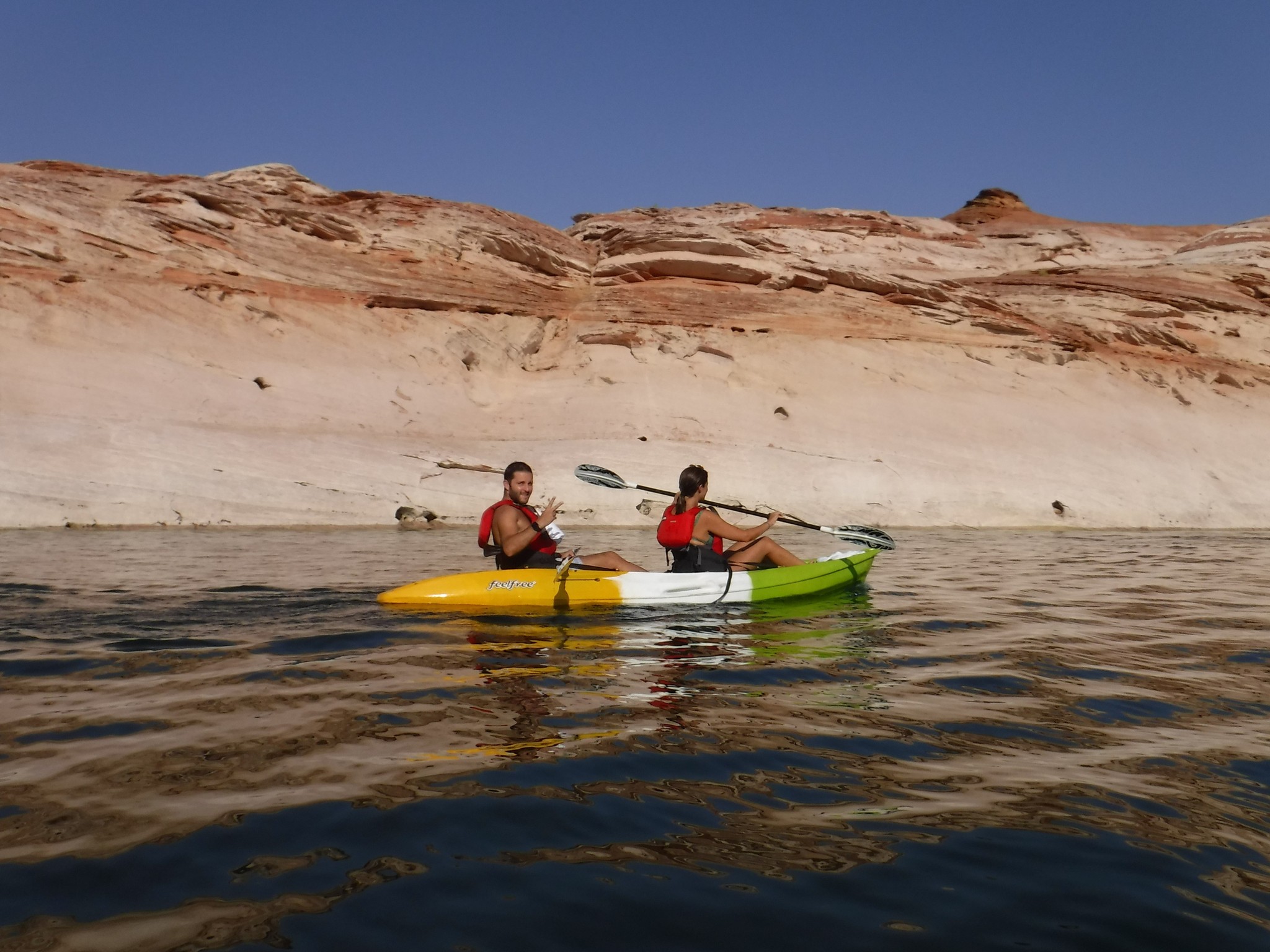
376,547 -> 884,617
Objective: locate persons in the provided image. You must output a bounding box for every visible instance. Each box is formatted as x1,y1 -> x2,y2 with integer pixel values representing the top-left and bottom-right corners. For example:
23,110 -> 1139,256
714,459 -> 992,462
657,464 -> 818,573
490,461 -> 650,572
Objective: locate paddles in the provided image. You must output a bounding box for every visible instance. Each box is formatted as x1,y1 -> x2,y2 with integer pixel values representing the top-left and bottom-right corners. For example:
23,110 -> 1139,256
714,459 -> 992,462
551,545 -> 581,585
572,462 -> 897,551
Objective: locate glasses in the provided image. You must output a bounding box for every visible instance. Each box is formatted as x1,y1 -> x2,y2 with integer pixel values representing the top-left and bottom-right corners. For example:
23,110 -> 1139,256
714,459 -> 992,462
690,464 -> 704,486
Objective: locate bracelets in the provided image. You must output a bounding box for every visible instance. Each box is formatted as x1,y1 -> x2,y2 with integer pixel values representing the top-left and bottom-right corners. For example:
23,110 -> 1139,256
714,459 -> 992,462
765,522 -> 770,529
531,521 -> 543,532
